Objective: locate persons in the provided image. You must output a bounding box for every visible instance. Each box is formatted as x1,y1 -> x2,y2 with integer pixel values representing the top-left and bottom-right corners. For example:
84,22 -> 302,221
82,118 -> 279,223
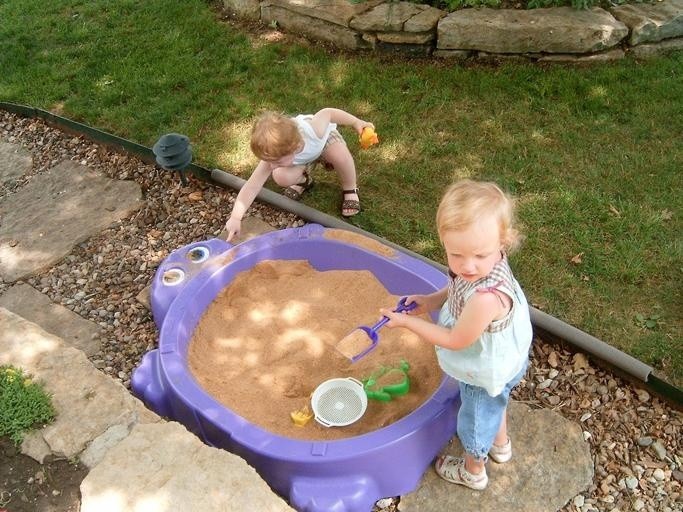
377,178 -> 534,492
222,107 -> 376,244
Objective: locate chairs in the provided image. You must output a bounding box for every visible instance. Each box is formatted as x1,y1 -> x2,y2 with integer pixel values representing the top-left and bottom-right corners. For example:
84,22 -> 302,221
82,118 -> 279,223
432,454 -> 490,492
489,434 -> 513,466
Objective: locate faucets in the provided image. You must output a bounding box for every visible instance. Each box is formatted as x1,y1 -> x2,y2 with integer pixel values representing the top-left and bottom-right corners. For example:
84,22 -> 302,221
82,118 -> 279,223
338,186 -> 361,218
282,170 -> 316,202
